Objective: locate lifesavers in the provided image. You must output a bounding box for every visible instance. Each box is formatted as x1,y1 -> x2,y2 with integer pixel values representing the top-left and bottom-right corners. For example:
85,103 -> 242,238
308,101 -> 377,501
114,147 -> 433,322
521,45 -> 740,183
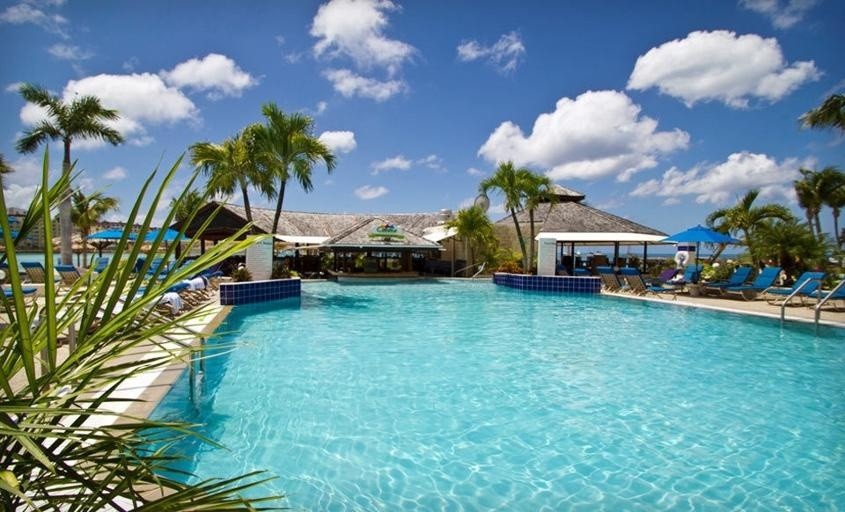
675,249 -> 689,266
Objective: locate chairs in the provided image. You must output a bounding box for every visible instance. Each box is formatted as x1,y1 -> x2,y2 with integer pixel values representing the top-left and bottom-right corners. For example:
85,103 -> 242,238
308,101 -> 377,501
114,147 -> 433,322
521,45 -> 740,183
1,253 -> 224,348
556,263 -> 845,311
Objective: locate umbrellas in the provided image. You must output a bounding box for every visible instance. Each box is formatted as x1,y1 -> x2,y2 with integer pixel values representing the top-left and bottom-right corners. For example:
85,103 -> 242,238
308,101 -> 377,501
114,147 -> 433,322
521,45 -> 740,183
143,227 -> 192,268
85,227 -> 143,263
658,223 -> 744,271
